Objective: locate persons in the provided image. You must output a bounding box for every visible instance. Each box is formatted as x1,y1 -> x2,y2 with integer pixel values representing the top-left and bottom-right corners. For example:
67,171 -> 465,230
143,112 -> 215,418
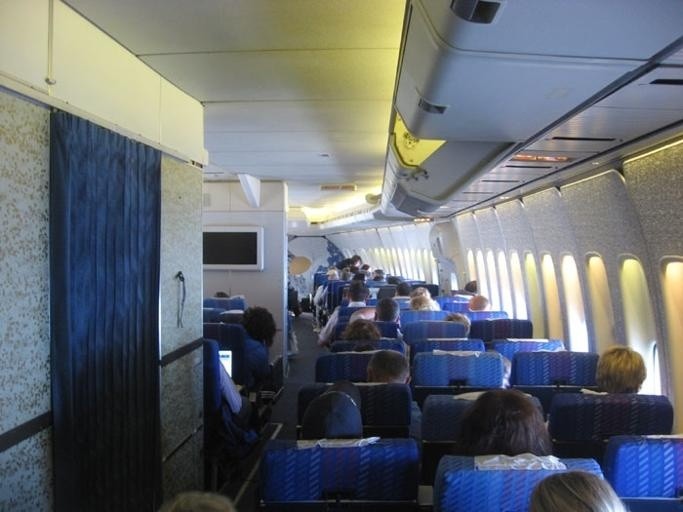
411,286 -> 431,298
312,269 -> 339,333
461,389 -> 551,454
370,269 -> 384,282
317,282 -> 370,347
591,346 -> 648,394
467,295 -> 491,312
240,305 -> 277,391
157,492 -> 237,511
352,255 -> 370,273
452,280 -> 478,295
340,268 -> 352,280
338,259 -> 352,269
526,471 -> 627,511
351,273 -> 368,285
219,356 -> 254,437
397,282 -> 410,296
362,350 -> 415,384
444,313 -> 471,335
349,297 -> 401,326
410,297 -> 436,311
338,318 -> 383,341
388,277 -> 401,284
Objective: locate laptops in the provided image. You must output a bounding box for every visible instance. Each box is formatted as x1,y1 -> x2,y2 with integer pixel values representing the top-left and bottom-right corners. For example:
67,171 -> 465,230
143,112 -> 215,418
218,349 -> 233,378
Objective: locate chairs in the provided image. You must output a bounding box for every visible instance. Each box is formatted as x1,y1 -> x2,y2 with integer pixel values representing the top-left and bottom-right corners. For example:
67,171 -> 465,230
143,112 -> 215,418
603,434 -> 683,511
314,266 -> 567,354
296,383 -> 412,438
549,392 -> 673,458
414,351 -> 505,410
420,394 -> 551,446
256,437 -> 423,510
417,452 -> 605,511
203,297 -> 283,462
510,351 -> 599,419
315,351 -> 410,383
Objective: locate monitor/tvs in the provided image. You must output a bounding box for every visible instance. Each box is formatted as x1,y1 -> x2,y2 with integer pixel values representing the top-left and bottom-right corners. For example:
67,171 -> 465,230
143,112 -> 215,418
202,225 -> 264,272
